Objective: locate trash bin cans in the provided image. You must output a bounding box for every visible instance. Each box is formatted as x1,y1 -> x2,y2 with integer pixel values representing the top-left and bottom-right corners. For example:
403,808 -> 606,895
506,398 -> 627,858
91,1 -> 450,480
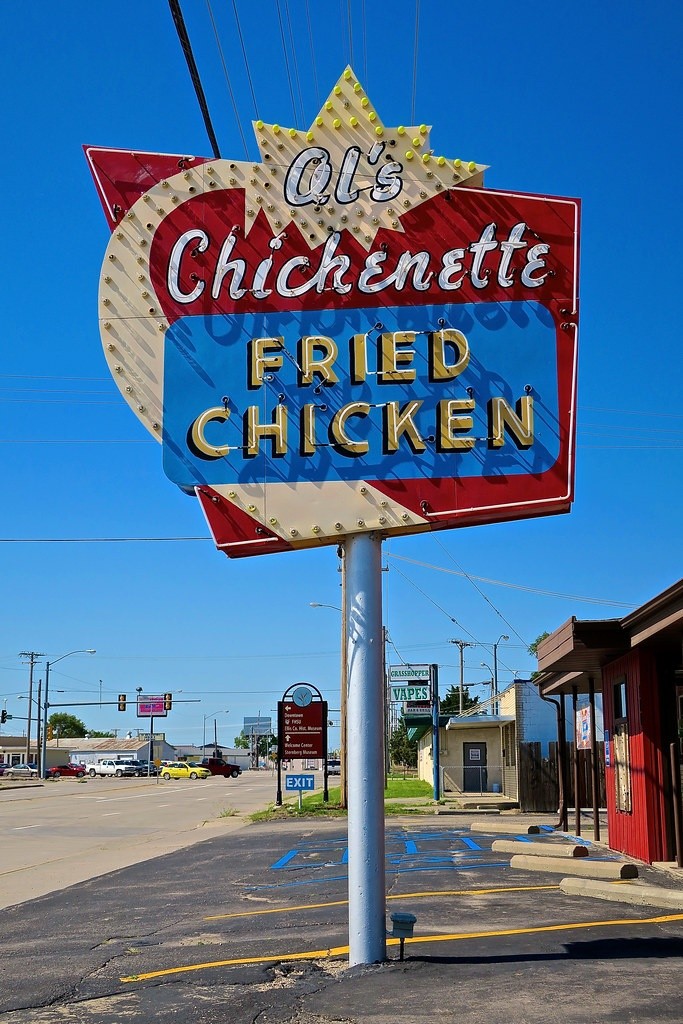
491,783 -> 501,793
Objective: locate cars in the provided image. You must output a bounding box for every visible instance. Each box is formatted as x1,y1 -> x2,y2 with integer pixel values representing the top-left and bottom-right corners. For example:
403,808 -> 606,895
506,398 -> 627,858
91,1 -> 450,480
0,763 -> 12,776
47,764 -> 86,778
160,761 -> 212,780
3,763 -> 51,779
327,759 -> 342,776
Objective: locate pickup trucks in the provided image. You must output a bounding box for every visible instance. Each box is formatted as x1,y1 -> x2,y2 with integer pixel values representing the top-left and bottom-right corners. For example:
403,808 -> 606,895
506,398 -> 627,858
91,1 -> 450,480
195,758 -> 242,779
122,758 -> 173,778
85,759 -> 137,778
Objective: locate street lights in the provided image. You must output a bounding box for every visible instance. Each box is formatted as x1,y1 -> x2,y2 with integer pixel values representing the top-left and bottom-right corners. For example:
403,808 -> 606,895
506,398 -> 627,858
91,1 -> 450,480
480,634 -> 510,716
18,649 -> 96,779
150,690 -> 182,762
202,710 -> 230,759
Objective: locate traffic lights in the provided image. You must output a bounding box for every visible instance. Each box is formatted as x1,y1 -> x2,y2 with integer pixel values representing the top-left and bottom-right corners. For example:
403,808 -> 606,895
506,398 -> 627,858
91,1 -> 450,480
1,710 -> 7,724
